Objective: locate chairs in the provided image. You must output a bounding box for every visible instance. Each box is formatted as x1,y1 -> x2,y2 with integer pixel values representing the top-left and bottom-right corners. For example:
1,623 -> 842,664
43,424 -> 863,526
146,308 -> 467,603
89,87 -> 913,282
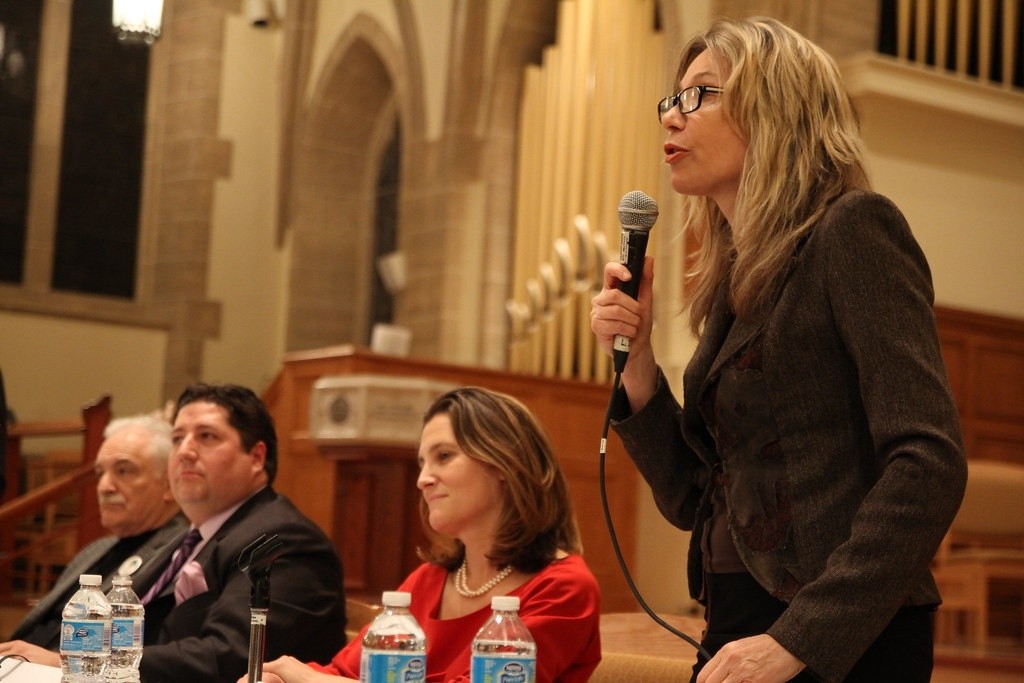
921,457 -> 1023,649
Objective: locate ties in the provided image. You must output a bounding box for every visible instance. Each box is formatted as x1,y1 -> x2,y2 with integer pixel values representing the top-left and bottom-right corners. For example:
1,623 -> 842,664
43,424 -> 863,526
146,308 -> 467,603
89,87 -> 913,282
140,527 -> 203,607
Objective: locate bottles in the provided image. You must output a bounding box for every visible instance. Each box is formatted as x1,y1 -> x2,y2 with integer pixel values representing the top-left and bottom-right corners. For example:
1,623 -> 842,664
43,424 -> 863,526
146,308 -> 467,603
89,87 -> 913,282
358,591 -> 428,683
104,575 -> 145,683
469,596 -> 536,683
59,574 -> 112,683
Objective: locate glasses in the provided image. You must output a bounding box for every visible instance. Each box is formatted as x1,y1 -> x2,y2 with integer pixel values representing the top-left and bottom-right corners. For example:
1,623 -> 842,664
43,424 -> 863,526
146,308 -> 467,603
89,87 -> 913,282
657,85 -> 726,123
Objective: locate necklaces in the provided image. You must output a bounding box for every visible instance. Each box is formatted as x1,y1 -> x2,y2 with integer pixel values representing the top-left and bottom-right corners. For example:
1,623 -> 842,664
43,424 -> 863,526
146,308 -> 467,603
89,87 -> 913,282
455,559 -> 515,597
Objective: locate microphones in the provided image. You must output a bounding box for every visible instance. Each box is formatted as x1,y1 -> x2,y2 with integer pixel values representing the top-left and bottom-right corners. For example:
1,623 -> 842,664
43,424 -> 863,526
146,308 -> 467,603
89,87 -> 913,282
614,191 -> 657,372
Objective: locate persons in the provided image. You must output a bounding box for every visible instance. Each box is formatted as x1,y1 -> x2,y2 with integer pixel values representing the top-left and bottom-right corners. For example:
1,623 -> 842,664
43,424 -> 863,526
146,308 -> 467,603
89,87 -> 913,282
0,381 -> 348,683
238,386 -> 602,683
589,17 -> 969,683
